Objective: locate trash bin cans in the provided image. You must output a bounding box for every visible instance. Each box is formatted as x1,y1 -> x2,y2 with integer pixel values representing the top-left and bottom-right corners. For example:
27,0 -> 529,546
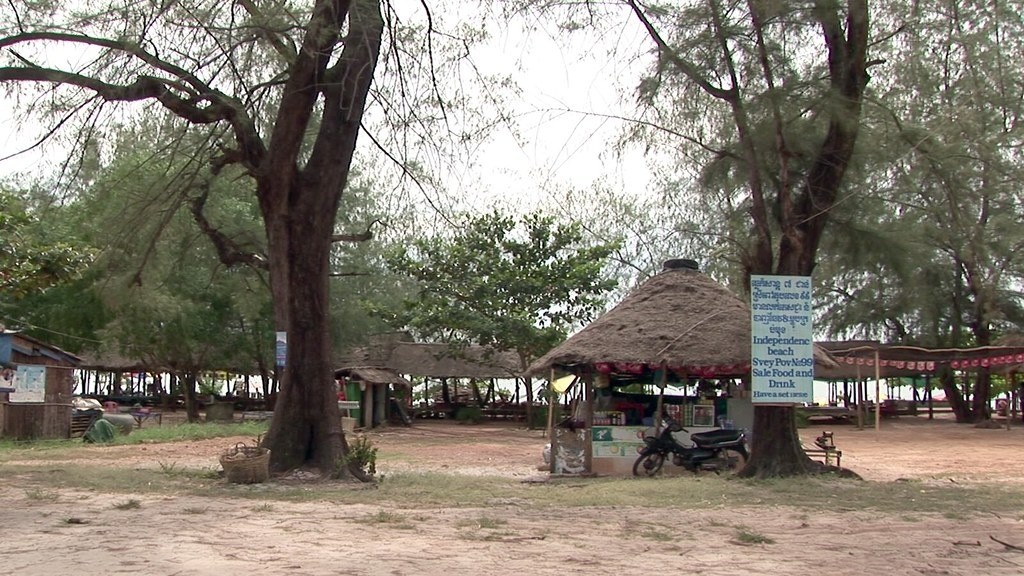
456,394 -> 469,420
885,399 -> 897,416
995,399 -> 1008,416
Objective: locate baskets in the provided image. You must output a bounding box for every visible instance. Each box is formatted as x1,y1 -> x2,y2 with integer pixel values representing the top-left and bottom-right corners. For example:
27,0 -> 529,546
644,436 -> 659,447
220,441 -> 272,483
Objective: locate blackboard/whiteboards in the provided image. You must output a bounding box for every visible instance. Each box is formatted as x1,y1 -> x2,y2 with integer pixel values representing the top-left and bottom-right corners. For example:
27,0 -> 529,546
692,405 -> 715,427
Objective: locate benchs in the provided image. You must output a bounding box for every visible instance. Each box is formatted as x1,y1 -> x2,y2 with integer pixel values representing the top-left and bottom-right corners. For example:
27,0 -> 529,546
799,442 -> 842,468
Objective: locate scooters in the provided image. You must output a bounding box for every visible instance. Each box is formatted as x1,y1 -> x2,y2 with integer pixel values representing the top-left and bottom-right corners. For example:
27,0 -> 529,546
632,405 -> 751,480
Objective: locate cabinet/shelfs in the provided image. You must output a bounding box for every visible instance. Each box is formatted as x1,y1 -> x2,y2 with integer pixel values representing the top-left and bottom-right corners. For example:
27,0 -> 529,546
693,405 -> 715,427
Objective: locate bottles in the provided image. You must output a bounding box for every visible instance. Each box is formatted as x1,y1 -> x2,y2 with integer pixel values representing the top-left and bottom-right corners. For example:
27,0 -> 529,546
612,414 -> 621,426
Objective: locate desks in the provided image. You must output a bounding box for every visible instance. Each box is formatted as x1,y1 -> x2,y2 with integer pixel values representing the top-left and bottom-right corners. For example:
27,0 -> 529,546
126,406 -> 163,431
796,406 -> 850,424
